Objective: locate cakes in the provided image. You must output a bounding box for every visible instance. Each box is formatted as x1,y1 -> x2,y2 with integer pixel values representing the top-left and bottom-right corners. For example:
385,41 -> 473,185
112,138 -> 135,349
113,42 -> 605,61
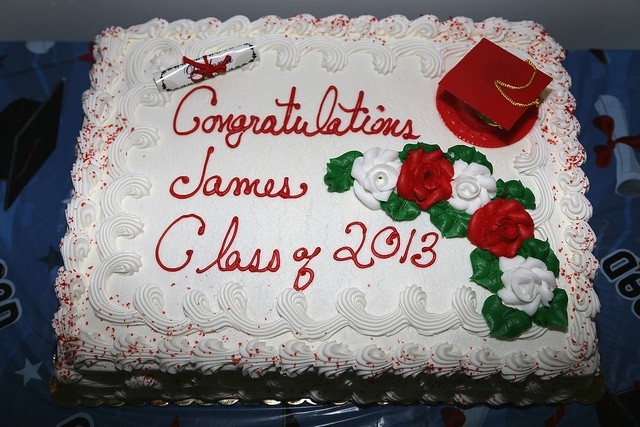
49,13 -> 605,406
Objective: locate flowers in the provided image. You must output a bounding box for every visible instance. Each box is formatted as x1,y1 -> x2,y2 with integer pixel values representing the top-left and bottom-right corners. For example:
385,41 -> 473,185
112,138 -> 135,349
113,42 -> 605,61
325,142 -> 568,339
